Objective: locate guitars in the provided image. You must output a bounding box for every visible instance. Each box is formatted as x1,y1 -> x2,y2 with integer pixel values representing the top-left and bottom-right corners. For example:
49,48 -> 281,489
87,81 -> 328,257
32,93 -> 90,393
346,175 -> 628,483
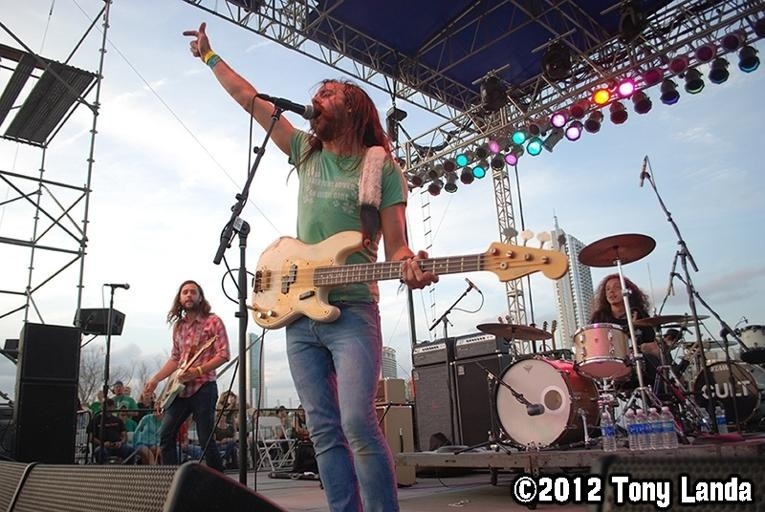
250,229 -> 572,330
155,332 -> 223,411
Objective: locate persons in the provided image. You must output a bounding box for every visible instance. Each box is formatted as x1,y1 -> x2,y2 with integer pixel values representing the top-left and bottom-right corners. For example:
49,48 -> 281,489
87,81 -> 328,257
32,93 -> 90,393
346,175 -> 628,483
183,22 -> 439,512
116,404 -> 135,446
144,280 -> 232,472
292,403 -> 308,439
139,390 -> 155,417
133,401 -> 165,464
89,391 -> 104,412
212,411 -> 236,465
274,405 -> 292,438
88,400 -> 126,461
586,275 -> 662,415
657,328 -> 691,386
108,383 -> 138,422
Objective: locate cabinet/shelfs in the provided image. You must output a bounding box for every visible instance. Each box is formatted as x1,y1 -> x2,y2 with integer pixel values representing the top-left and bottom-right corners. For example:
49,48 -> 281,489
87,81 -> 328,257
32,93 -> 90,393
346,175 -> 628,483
409,353 -> 511,451
374,405 -> 416,488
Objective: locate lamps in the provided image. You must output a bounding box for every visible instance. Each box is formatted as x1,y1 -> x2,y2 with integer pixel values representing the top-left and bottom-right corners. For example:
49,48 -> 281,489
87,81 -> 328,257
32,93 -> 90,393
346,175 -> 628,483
443,172 -> 460,194
476,142 -> 490,160
442,158 -> 457,174
551,110 -> 570,128
527,137 -> 542,156
473,158 -> 491,179
543,127 -> 565,152
685,67 -> 704,94
488,134 -> 509,154
459,167 -> 474,185
427,179 -> 444,195
415,169 -> 428,188
480,77 -> 507,110
510,131 -> 527,145
490,154 -> 505,173
738,46 -> 760,73
707,58 -> 730,84
659,78 -> 680,105
644,67 -> 664,84
669,55 -> 689,74
544,43 -> 571,79
634,92 -> 651,114
614,78 -> 637,98
455,150 -> 474,166
505,146 -> 525,167
720,29 -> 748,52
694,44 -> 717,64
592,87 -> 612,107
619,7 -> 650,46
528,117 -> 550,136
429,165 -> 444,178
566,120 -> 584,141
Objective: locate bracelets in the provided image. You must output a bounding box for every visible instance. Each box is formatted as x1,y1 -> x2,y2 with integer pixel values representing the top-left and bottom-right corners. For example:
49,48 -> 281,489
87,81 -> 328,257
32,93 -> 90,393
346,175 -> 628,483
204,52 -> 221,65
197,366 -> 204,378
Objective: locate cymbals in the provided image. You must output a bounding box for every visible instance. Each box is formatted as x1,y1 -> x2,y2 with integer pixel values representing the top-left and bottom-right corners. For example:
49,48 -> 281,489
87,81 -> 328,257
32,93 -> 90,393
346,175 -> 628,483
630,311 -> 714,332
680,339 -> 738,350
576,232 -> 660,270
475,315 -> 560,349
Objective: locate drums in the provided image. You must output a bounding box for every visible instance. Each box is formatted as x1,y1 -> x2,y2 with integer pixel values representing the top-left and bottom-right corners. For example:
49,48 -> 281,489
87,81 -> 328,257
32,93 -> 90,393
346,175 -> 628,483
692,359 -> 765,433
568,320 -> 632,383
736,324 -> 765,364
488,349 -> 606,447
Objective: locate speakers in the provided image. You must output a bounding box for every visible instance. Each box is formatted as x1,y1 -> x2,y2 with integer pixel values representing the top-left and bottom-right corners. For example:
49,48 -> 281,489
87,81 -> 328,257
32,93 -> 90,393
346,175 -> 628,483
5,339 -> 19,359
410,340 -> 455,451
13,323 -> 82,464
74,308 -> 126,336
454,333 -> 512,449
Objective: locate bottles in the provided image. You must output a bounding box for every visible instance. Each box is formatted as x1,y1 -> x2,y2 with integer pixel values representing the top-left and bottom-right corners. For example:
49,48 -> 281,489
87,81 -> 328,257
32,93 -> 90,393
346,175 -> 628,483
626,409 -> 639,450
637,409 -> 648,451
601,413 -> 618,453
715,405 -> 729,437
648,407 -> 661,450
661,406 -> 677,449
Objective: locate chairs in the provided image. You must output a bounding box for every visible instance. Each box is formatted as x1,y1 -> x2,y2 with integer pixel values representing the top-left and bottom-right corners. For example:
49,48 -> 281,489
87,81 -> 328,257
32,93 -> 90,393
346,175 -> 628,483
608,100 -> 628,125
571,99 -> 590,119
256,415 -> 295,473
584,111 -> 604,134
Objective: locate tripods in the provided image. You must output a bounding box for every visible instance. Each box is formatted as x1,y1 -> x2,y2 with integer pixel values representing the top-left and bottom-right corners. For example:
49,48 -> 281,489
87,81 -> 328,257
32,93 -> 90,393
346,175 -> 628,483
654,326 -> 713,445
596,260 -> 689,446
451,384 -> 523,455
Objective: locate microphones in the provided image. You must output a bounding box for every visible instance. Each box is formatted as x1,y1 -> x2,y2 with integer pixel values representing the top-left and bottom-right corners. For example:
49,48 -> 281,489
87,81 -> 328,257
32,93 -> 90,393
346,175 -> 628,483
257,94 -> 317,120
104,283 -> 130,289
640,156 -> 650,187
464,277 -> 483,295
527,404 -> 545,416
